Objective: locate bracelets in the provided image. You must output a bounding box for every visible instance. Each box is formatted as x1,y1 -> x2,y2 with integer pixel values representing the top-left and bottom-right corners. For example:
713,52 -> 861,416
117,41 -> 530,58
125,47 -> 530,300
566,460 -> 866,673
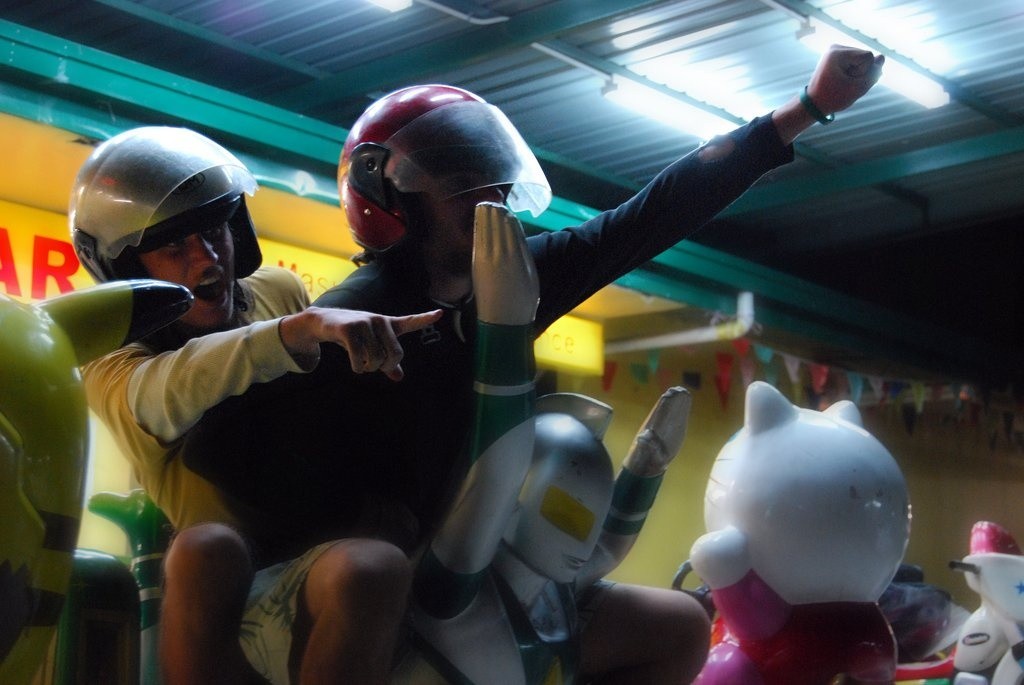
800,86 -> 835,125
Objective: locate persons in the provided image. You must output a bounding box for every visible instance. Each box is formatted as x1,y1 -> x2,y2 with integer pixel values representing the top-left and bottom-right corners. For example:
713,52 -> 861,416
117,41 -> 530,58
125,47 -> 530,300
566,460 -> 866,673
69,127 -> 443,685
387,200 -> 692,685
182,44 -> 884,685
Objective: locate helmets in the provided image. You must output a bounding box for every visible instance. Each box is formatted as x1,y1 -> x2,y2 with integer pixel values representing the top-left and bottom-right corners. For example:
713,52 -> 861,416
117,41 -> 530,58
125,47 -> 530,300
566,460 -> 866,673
71,125 -> 262,284
879,565 -> 951,664
337,84 -> 521,255
